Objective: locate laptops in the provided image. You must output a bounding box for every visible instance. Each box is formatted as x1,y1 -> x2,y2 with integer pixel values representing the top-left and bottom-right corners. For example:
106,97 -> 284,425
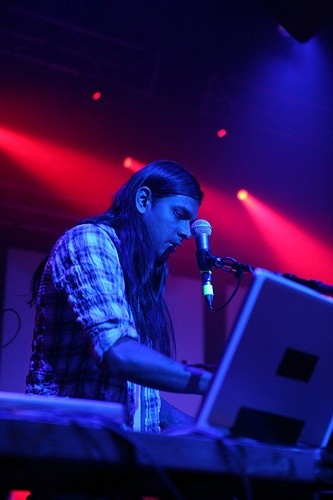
166,270 -> 333,451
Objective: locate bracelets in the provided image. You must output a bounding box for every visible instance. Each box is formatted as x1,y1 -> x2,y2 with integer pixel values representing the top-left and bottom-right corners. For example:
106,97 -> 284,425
183,366 -> 205,395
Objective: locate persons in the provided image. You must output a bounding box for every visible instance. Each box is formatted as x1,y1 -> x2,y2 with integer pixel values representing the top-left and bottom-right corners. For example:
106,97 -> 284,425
27,161 -> 221,435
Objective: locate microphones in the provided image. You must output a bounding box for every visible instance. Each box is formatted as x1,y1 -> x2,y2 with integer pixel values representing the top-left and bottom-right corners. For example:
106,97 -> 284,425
191,219 -> 214,308
